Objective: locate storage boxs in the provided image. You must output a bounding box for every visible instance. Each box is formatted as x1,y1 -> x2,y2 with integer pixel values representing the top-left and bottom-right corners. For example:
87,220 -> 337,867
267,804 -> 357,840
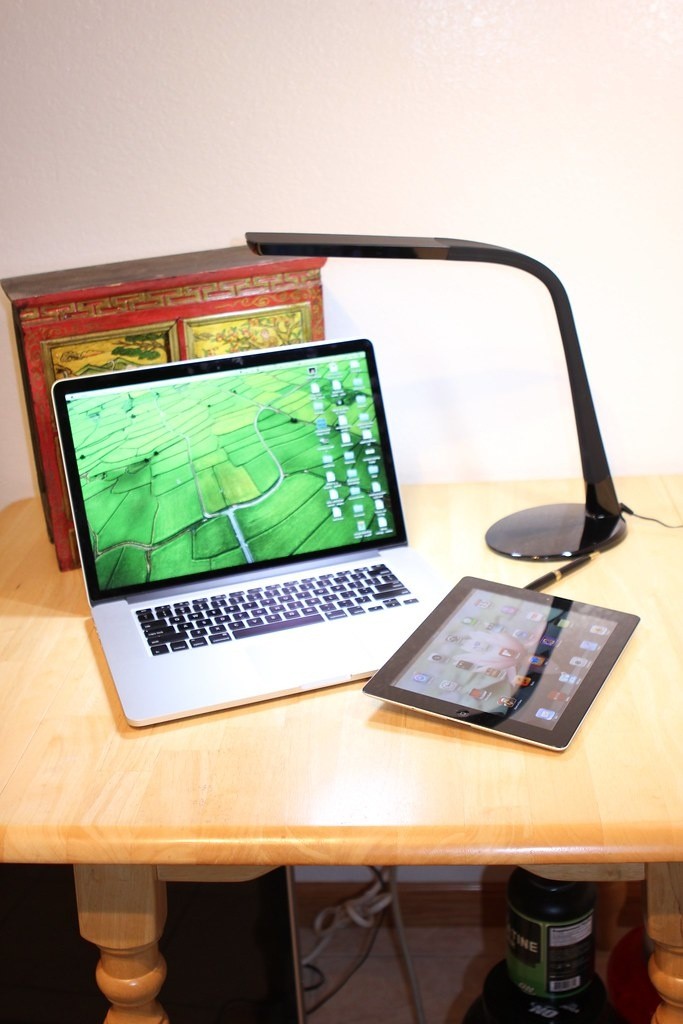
0,246 -> 327,567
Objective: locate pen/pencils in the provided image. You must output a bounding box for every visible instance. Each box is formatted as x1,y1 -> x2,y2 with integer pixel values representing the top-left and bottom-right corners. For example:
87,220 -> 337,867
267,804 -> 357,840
525,551 -> 602,591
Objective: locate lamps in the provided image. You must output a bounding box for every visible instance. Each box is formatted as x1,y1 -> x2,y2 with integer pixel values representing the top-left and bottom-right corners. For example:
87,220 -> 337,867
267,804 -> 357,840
245,232 -> 683,562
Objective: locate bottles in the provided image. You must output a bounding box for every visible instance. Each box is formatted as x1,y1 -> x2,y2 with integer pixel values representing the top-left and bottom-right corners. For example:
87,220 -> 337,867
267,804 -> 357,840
459,867 -> 633,1024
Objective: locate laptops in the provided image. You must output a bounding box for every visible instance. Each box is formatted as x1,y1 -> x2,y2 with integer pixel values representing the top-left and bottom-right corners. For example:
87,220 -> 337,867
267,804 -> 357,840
50,339 -> 454,727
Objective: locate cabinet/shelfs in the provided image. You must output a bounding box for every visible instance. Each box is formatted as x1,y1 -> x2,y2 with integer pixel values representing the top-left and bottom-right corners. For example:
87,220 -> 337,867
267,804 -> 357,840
0,472 -> 683,1023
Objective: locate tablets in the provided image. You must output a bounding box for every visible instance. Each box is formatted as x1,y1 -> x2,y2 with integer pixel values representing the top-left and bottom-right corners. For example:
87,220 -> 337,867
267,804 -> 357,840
361,576 -> 640,752
